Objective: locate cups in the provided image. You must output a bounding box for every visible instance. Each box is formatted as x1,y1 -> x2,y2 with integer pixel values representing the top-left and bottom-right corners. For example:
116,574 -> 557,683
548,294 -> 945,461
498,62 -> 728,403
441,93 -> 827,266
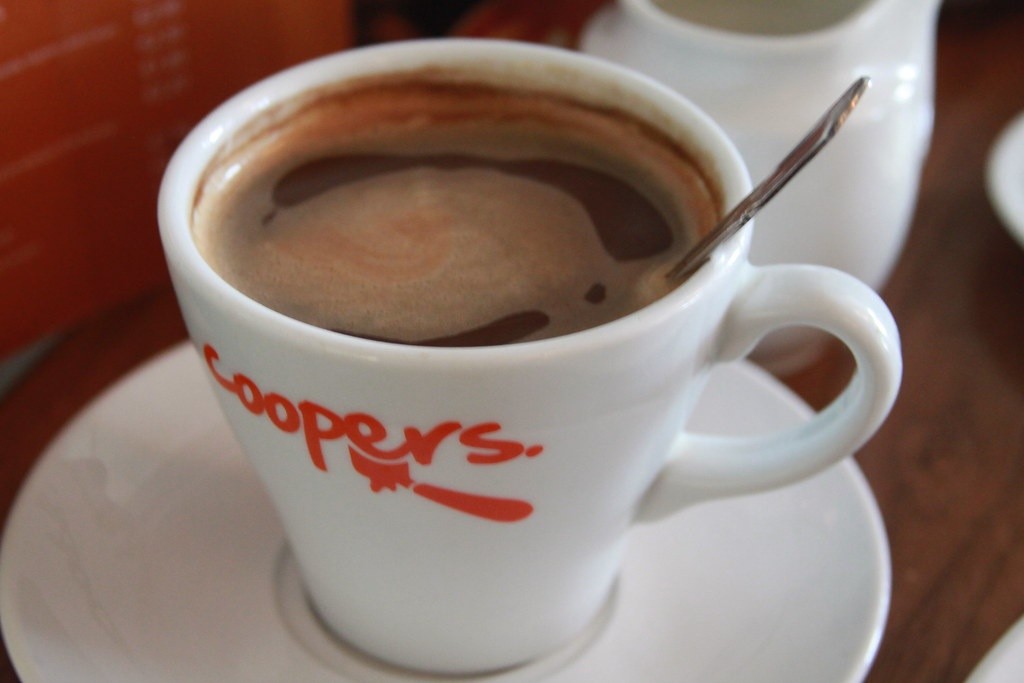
159,38 -> 903,673
579,0 -> 941,365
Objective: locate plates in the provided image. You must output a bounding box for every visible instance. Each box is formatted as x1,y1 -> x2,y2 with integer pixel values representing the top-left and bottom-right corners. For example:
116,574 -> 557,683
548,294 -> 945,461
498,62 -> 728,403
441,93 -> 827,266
0,341 -> 892,683
